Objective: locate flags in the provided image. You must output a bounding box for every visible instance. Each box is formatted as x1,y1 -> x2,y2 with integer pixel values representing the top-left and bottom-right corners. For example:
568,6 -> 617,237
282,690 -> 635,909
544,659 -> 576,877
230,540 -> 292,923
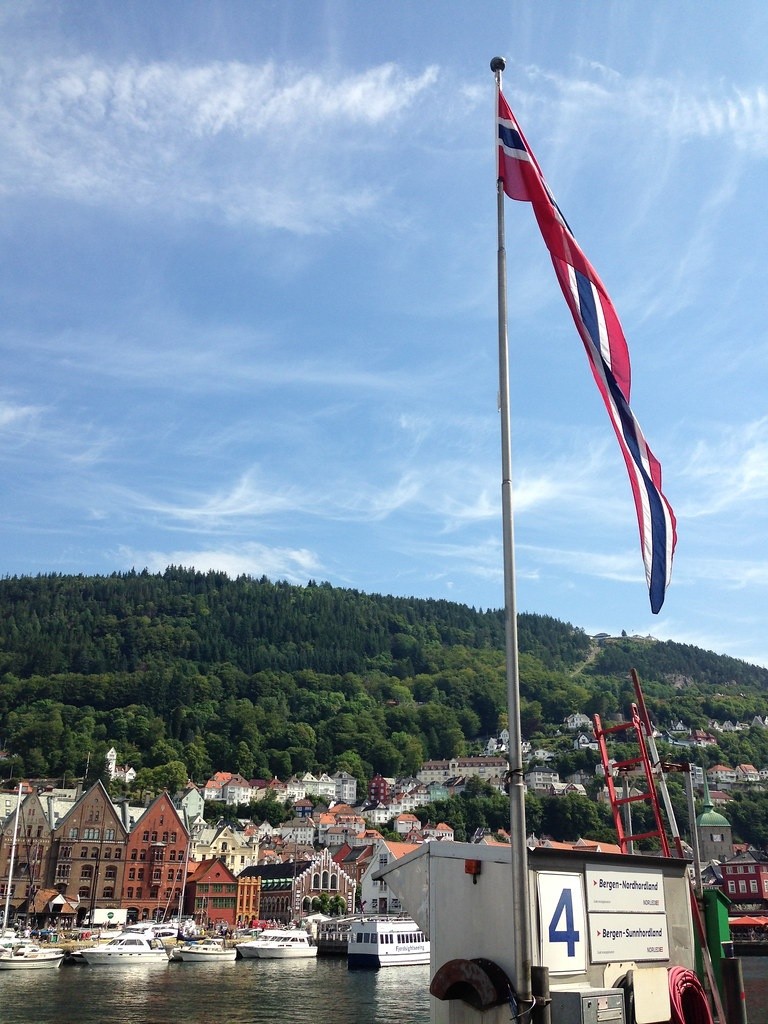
496,89 -> 678,615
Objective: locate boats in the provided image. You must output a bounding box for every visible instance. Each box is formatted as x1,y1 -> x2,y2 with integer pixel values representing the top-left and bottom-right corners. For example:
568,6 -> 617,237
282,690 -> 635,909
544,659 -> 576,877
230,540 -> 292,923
256,930 -> 318,959
0,944 -> 64,969
235,930 -> 278,957
177,942 -> 237,961
70,944 -> 104,962
80,934 -> 169,964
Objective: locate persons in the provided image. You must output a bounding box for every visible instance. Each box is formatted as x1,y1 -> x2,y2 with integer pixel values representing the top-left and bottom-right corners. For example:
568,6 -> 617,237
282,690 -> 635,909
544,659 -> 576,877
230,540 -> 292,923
13,917 -> 300,943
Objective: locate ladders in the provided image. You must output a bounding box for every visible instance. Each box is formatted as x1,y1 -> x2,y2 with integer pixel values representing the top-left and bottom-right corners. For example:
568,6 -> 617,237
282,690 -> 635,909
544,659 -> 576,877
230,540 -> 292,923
593,703 -> 672,859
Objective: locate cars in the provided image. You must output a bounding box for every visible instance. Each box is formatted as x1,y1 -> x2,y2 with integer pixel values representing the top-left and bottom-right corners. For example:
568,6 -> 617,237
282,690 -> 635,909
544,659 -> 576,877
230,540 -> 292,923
115,923 -> 178,940
